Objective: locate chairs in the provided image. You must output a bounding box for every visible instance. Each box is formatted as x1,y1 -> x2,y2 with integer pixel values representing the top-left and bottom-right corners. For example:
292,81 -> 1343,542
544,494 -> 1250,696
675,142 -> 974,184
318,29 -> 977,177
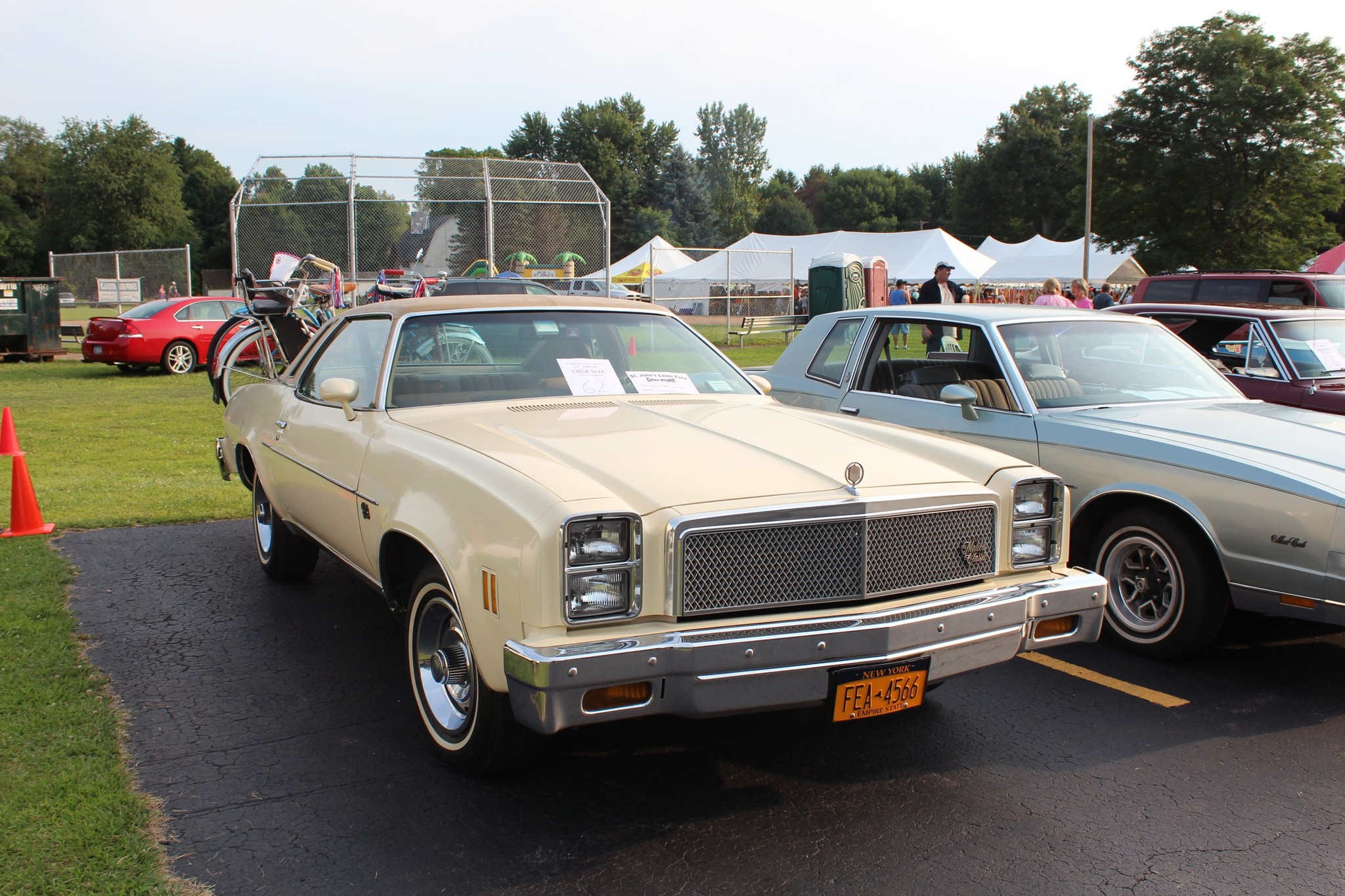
193,305 -> 226,319
240,268 -> 295,316
898,364 -> 1082,411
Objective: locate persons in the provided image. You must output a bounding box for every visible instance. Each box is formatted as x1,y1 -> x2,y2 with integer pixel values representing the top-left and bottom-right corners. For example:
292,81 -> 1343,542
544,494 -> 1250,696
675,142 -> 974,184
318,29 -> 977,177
714,261 -> 1136,360
159,281 -> 181,299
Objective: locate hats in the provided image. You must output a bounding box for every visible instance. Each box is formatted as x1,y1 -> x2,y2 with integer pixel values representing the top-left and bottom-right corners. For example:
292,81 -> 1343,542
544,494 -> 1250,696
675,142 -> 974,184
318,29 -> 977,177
935,261 -> 955,269
897,279 -> 907,286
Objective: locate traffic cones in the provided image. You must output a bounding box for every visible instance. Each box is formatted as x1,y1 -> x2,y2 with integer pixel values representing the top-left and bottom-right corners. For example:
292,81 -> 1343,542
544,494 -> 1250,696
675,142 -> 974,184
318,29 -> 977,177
0,407 -> 27,457
627,335 -> 639,355
0,454 -> 57,538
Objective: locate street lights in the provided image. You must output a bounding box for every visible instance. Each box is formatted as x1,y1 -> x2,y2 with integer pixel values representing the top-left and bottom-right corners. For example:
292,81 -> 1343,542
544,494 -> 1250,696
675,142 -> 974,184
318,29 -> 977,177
1083,114 -> 1114,283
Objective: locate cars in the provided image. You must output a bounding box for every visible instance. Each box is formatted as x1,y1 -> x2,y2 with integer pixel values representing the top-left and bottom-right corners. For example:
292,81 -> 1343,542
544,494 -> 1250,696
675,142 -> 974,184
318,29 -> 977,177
58,293 -> 76,307
80,296 -> 310,375
213,294 -> 1112,780
925,301 -> 1345,415
87,290 -> 119,309
740,303 -> 1344,662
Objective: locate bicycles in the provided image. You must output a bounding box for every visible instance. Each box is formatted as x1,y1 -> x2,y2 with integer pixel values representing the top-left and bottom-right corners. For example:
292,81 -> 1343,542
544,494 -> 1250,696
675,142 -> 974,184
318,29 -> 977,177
206,248 -> 448,409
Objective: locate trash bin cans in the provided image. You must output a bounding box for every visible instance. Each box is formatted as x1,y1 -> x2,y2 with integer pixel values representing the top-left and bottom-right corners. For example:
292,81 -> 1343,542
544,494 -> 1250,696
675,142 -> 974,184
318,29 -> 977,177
0,276 -> 62,363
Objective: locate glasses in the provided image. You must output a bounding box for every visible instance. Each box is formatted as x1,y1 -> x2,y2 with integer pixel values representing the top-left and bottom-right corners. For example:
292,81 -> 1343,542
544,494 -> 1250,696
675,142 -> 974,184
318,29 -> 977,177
1071,285 -> 1079,289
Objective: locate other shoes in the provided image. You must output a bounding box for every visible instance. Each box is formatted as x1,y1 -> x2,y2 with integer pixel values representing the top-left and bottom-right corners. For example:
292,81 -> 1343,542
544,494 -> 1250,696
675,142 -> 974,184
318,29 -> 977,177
894,347 -> 899,349
903,346 -> 910,349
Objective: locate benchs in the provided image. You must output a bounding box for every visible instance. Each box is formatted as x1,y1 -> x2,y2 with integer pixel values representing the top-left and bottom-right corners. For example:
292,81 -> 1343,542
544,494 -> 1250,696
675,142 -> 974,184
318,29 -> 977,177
729,315 -> 809,349
821,359 -> 995,396
60,325 -> 86,344
349,338 -> 590,409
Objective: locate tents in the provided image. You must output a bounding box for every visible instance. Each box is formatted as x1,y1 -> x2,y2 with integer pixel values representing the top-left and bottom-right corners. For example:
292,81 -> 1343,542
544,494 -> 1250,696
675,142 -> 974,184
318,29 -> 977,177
585,232 -> 1148,316
1300,243 -> 1345,311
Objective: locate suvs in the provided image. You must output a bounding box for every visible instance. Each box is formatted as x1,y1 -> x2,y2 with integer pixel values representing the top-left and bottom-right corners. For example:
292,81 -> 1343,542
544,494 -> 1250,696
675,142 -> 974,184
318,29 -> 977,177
428,277 -> 562,298
1131,269 -> 1345,312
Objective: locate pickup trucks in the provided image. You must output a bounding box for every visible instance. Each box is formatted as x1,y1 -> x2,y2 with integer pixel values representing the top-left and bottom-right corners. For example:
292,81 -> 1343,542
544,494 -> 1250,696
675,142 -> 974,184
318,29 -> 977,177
552,279 -> 651,303
